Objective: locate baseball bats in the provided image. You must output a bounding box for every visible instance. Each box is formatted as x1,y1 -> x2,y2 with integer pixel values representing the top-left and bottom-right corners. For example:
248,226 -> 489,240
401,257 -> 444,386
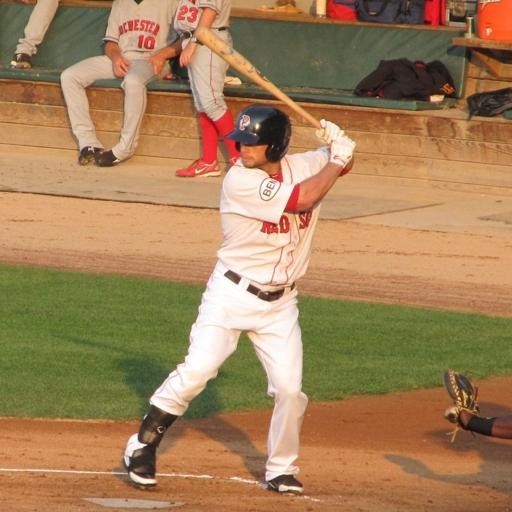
192,24 -> 353,172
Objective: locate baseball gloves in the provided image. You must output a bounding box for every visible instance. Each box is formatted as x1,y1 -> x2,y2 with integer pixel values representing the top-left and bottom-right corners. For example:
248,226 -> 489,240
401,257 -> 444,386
442,371 -> 479,430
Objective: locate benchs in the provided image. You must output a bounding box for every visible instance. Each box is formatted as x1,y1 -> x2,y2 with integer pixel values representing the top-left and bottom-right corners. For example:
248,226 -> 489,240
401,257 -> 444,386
0,4 -> 471,111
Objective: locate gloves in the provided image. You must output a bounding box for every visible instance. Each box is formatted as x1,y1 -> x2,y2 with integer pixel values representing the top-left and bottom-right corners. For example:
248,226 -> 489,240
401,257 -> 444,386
315,119 -> 356,169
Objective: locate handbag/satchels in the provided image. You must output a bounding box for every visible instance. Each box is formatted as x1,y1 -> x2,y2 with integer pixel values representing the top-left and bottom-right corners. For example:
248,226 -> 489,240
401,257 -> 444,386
354,58 -> 456,100
326,0 -> 425,24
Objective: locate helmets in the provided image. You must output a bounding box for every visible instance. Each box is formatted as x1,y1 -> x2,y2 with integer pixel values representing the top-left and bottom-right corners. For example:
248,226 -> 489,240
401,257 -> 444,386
225,105 -> 291,162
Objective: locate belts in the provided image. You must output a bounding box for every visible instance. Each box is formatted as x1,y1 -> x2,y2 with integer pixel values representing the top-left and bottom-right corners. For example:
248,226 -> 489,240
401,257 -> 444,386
224,270 -> 295,301
181,28 -> 225,38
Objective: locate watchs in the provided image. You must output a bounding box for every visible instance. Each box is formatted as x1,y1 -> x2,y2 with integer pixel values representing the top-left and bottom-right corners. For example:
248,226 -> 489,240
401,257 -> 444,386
189,34 -> 204,46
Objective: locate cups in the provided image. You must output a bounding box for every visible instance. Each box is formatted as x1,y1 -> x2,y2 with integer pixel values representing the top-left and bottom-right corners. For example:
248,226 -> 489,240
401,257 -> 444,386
315,0 -> 327,18
465,16 -> 477,39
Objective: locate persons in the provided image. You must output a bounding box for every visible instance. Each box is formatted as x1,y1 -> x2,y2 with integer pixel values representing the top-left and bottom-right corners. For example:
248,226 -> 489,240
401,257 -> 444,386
172,0 -> 241,179
445,367 -> 512,440
60,1 -> 177,167
9,1 -> 62,69
118,107 -> 356,495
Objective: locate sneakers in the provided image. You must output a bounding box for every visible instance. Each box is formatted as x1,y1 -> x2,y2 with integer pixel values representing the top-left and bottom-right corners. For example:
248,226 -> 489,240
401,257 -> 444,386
124,433 -> 158,488
176,158 -> 221,177
79,147 -> 120,167
10,53 -> 31,69
268,475 -> 304,494
